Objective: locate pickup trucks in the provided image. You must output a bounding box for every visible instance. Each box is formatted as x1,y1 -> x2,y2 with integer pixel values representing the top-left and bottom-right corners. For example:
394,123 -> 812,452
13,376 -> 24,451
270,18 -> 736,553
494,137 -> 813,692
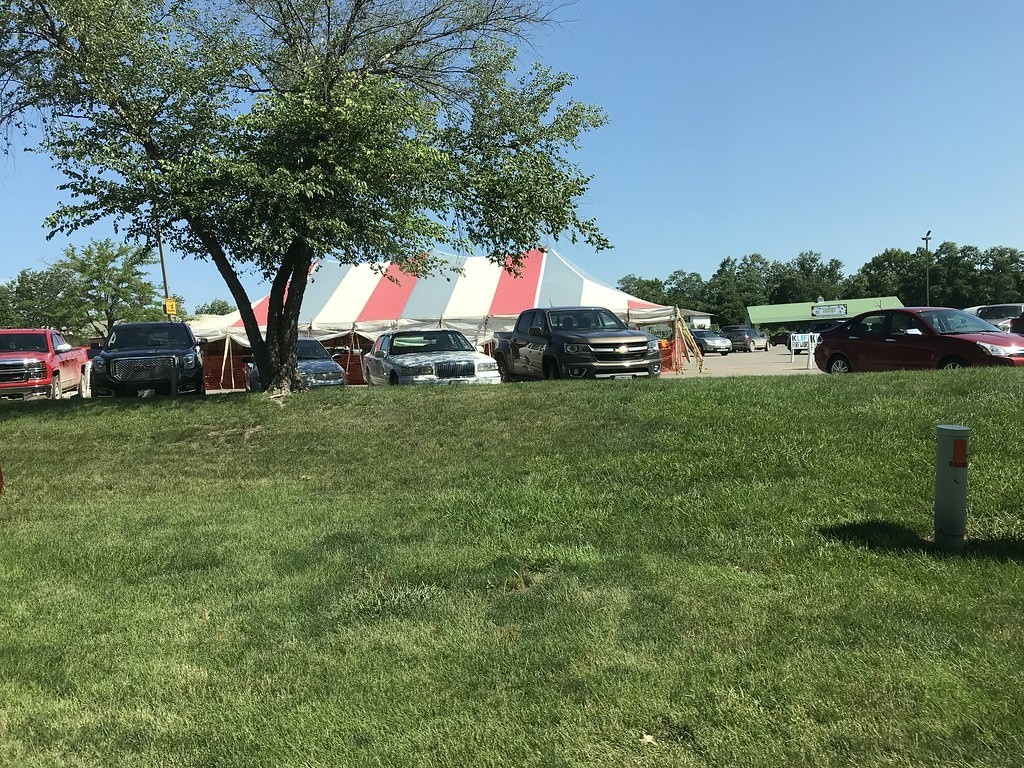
0,329 -> 92,403
494,306 -> 662,383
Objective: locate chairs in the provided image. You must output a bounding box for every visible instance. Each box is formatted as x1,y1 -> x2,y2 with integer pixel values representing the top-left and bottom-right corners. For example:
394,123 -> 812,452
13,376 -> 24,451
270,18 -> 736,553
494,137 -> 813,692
435,335 -> 451,350
563,317 -> 575,331
577,317 -> 591,328
859,323 -> 869,331
923,315 -> 934,325
870,323 -> 882,329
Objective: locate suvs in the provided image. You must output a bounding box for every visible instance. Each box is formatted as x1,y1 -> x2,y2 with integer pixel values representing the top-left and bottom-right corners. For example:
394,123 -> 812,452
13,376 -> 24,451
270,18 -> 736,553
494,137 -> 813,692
718,325 -> 750,340
86,322 -> 209,400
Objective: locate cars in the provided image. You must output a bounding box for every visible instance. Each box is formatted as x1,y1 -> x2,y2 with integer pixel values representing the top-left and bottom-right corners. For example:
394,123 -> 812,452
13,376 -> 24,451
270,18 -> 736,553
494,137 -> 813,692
726,331 -> 770,352
815,307 -> 1024,374
362,328 -> 502,388
689,329 -> 732,356
242,336 -> 347,392
770,331 -> 794,346
